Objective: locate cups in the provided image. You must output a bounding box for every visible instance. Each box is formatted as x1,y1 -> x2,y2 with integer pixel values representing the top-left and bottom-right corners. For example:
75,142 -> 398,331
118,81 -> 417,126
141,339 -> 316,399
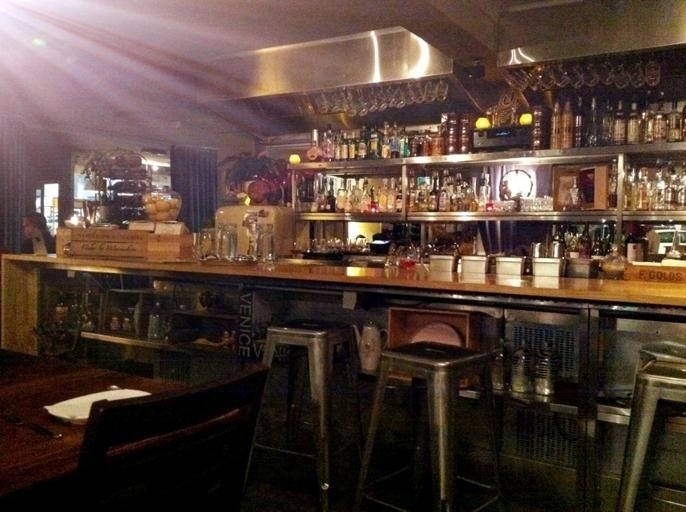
82,199 -> 97,228
291,81 -> 450,117
502,60 -> 663,93
197,223 -> 279,271
305,235 -> 368,255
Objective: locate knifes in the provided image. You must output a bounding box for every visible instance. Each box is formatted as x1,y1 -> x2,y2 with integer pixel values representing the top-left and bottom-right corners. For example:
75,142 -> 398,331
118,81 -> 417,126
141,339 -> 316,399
2,411 -> 64,442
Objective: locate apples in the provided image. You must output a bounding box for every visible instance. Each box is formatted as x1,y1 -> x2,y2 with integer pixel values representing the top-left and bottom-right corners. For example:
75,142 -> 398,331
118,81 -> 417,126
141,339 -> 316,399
248,180 -> 271,204
266,180 -> 283,204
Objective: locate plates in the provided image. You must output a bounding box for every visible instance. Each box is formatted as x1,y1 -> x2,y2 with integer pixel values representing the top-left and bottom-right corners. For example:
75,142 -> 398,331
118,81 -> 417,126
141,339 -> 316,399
410,322 -> 462,348
43,389 -> 153,423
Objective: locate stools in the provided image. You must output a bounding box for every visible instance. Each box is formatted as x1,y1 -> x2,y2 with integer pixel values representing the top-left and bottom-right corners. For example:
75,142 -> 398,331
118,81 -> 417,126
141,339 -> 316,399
237,320 -> 364,511
354,341 -> 509,511
616,347 -> 686,512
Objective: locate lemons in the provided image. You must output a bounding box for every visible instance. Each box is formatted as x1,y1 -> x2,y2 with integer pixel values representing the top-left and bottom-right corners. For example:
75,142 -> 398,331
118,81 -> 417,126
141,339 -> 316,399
519,113 -> 533,125
475,117 -> 490,129
289,154 -> 301,165
142,195 -> 182,223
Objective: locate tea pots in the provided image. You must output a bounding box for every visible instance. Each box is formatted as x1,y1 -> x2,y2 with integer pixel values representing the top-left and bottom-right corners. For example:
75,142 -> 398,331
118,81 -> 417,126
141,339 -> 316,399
350,320 -> 391,373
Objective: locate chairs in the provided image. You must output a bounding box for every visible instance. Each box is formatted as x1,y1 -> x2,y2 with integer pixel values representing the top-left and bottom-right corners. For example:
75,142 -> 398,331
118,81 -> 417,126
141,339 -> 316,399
67,361 -> 269,511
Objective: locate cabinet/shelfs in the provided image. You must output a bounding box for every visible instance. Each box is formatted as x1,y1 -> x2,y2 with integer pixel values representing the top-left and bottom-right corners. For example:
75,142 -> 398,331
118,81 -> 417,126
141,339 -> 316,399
37,284 -> 252,385
289,140 -> 686,268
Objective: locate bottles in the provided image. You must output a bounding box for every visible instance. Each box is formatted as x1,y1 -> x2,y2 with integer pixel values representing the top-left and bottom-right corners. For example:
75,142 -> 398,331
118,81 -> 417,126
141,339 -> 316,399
55,289 -> 183,343
528,241 -> 544,258
548,239 -> 566,258
395,222 -> 419,269
553,221 -> 684,280
486,335 -> 557,397
296,111 -> 491,216
564,168 -> 588,211
528,88 -> 686,150
607,157 -> 686,212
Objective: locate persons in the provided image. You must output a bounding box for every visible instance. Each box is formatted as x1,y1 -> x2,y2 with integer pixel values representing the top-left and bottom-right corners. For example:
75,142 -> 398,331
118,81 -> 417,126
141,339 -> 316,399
20,212 -> 56,253
